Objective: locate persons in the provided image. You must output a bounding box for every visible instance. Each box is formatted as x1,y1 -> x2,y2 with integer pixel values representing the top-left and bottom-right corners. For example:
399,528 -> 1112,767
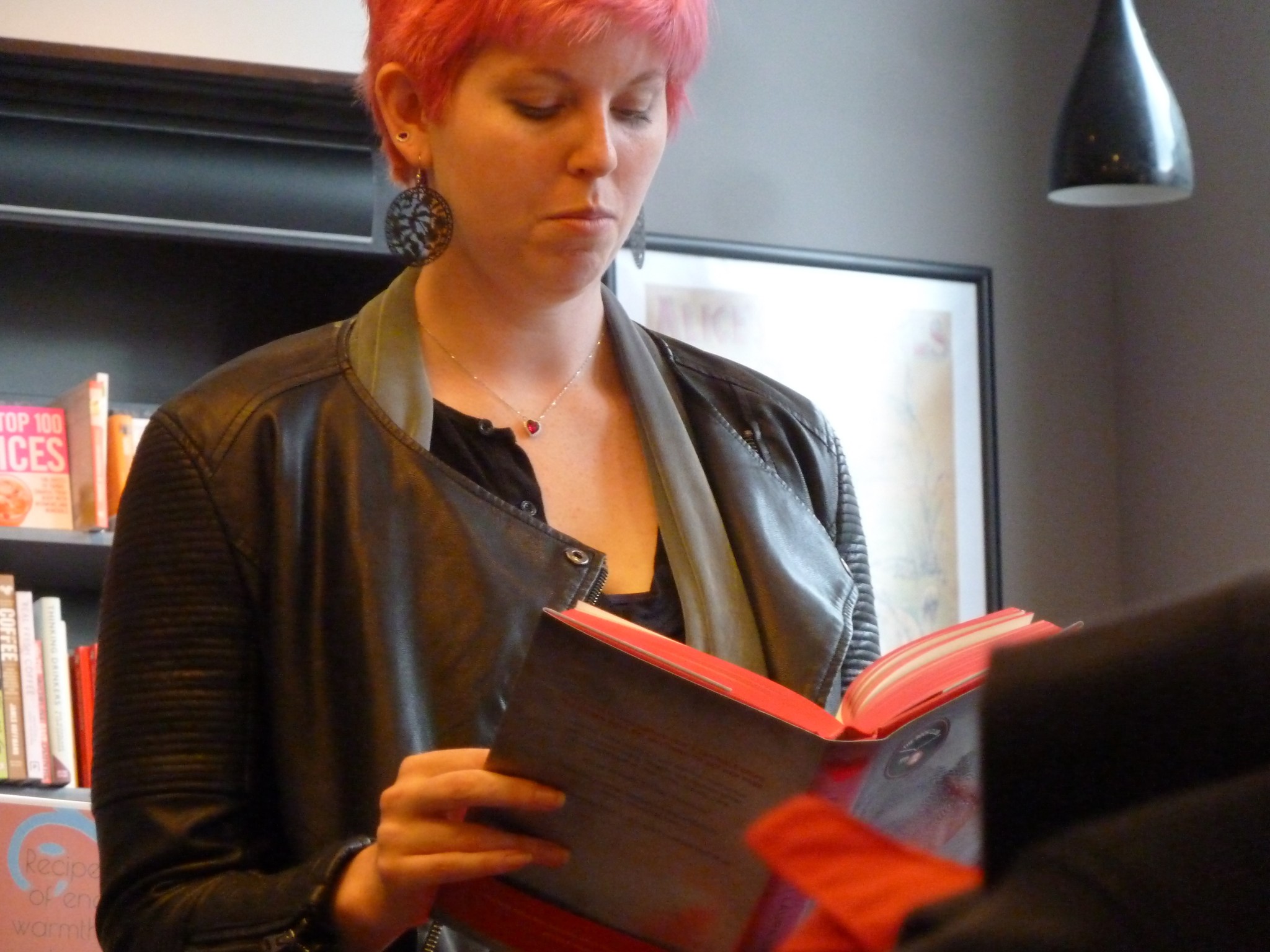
91,1 -> 895,950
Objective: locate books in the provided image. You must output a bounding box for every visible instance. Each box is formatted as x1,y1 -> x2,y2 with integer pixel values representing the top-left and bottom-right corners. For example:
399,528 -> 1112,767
419,596 -> 1084,950
1,375 -> 164,536
2,572 -> 101,796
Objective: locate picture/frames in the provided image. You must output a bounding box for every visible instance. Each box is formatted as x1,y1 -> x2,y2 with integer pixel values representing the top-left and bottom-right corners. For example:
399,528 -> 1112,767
611,218 -> 1000,874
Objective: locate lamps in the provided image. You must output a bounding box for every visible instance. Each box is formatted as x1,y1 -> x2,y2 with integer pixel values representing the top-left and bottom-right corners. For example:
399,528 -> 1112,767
1045,1 -> 1195,207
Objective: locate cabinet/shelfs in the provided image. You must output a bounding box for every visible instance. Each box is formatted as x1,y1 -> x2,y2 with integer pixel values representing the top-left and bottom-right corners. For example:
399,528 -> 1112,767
0,82 -> 420,805
